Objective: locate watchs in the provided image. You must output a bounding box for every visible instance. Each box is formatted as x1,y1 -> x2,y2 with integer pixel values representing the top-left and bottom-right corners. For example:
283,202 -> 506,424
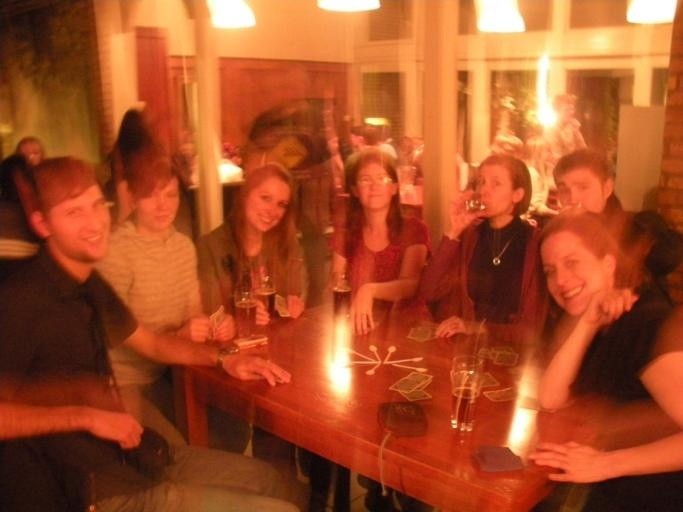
216,341 -> 239,369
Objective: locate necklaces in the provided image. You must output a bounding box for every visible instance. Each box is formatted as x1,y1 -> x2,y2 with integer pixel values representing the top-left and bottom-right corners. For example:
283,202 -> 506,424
484,227 -> 523,266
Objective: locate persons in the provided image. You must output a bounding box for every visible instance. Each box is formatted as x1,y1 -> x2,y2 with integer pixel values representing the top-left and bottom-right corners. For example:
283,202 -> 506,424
326,151 -> 432,336
15,136 -> 45,167
418,154 -> 552,346
526,212 -> 682,485
94,156 -> 237,446
554,94 -> 588,156
0,156 -> 314,512
350,125 -> 381,151
109,108 -> 155,225
197,161 -> 310,327
553,148 -> 682,300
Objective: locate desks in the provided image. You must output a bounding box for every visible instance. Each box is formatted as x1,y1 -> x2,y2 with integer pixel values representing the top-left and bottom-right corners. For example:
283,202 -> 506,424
169,305 -> 626,512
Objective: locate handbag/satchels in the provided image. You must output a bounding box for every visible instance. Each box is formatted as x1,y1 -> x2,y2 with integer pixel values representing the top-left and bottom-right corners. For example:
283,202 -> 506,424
377,402 -> 427,436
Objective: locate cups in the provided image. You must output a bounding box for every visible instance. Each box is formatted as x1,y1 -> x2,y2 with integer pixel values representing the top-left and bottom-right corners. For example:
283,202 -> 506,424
396,166 -> 417,192
332,272 -> 353,323
449,355 -> 485,432
465,191 -> 490,212
234,287 -> 259,338
256,272 -> 277,323
